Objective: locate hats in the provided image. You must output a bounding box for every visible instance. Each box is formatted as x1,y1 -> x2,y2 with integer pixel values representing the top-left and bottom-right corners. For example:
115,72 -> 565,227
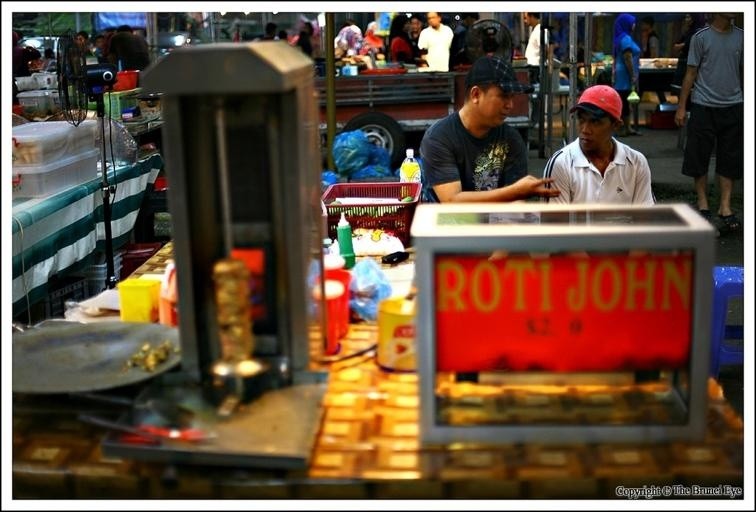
571,86 -> 623,121
463,55 -> 534,94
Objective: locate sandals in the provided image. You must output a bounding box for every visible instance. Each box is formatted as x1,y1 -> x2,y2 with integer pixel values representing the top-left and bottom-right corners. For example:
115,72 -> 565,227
617,130 -> 643,138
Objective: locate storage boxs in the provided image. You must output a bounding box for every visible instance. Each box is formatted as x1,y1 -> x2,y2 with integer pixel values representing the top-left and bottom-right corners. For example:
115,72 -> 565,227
104,88 -> 143,120
12,120 -> 98,167
39,276 -> 90,320
85,252 -> 124,298
12,149 -> 101,198
319,181 -> 423,247
17,91 -> 53,112
645,109 -> 678,129
121,242 -> 162,280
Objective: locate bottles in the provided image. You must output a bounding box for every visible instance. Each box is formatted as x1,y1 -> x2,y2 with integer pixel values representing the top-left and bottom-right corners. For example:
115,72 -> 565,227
400,149 -> 421,199
337,212 -> 356,270
376,298 -> 417,372
375,54 -> 387,69
322,238 -> 332,255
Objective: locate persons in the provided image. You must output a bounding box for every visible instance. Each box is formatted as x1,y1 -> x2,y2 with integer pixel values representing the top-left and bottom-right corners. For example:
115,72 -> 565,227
77,33 -> 90,61
389,15 -> 429,66
613,14 -> 641,136
418,12 -> 455,71
449,13 -> 480,73
13,34 -> 40,105
109,24 -> 151,89
409,16 -> 424,51
361,21 -> 383,58
675,13 -> 743,230
541,85 -> 654,204
300,23 -> 320,64
675,14 -> 700,129
420,56 -> 560,204
641,16 -> 660,93
262,24 -> 274,45
28,48 -> 42,70
524,12 -> 551,126
93,35 -> 107,63
44,49 -> 56,70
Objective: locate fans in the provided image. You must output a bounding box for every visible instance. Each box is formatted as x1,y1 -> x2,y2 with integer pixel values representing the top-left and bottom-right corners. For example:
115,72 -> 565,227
466,20 -> 514,68
56,26 -> 118,288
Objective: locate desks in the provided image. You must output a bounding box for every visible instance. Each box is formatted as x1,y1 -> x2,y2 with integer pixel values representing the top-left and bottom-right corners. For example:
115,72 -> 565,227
12,152 -> 161,304
12,239 -> 744,500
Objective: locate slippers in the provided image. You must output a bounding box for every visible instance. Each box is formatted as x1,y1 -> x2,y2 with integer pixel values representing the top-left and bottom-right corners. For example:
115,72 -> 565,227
700,209 -> 712,221
721,213 -> 740,229
